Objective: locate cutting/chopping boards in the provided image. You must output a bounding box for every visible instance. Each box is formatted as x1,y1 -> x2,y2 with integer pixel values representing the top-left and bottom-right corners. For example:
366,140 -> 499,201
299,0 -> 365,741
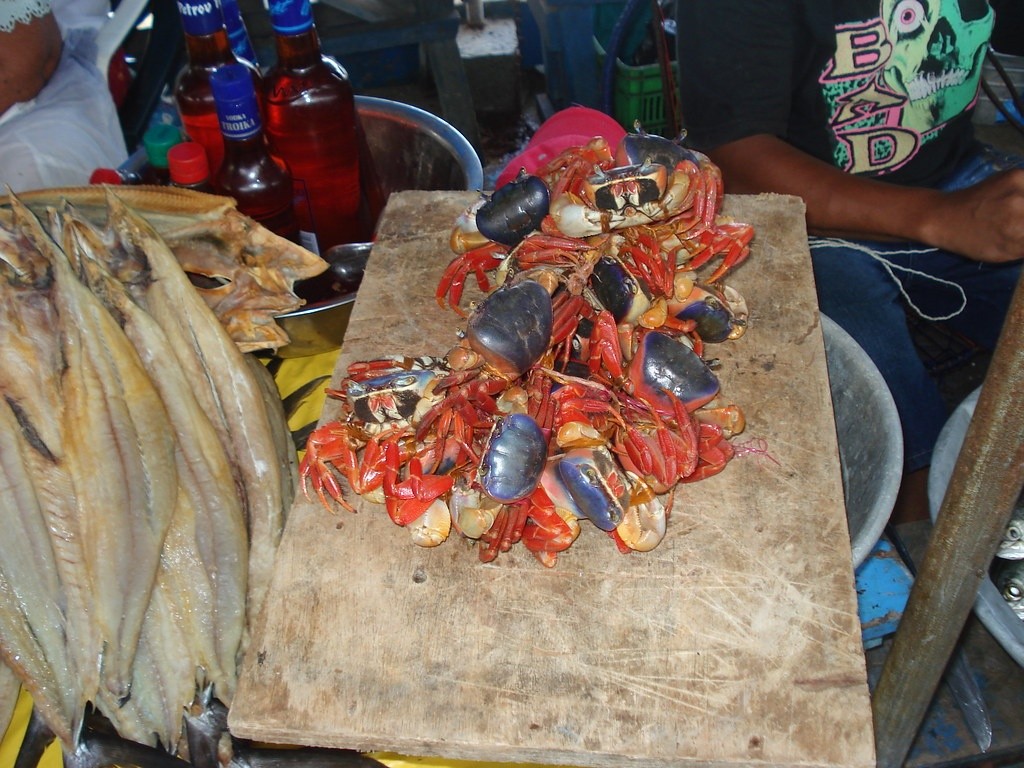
228,189 -> 878,768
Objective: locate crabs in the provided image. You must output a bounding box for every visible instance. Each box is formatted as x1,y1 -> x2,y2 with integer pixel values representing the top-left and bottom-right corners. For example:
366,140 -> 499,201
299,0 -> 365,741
299,119 -> 754,568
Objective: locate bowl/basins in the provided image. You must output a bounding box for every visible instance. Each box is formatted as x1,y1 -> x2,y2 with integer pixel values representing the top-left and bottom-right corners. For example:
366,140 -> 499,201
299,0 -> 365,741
927,384 -> 1024,670
818,310 -> 903,571
274,93 -> 485,359
275,242 -> 372,359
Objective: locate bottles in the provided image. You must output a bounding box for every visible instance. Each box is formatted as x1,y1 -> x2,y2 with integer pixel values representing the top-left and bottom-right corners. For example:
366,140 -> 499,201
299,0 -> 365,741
90,0 -> 383,305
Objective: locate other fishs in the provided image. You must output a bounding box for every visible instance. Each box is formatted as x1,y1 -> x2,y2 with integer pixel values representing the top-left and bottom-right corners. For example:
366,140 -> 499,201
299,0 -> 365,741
996,482 -> 1024,621
0,183 -> 389,767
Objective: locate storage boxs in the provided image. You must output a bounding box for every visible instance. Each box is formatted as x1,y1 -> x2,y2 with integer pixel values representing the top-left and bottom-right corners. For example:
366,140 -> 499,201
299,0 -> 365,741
591,26 -> 682,139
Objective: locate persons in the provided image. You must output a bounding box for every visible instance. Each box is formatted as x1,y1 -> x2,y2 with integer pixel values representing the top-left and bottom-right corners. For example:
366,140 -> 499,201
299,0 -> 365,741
0,0 -> 129,197
674,0 -> 1024,477
